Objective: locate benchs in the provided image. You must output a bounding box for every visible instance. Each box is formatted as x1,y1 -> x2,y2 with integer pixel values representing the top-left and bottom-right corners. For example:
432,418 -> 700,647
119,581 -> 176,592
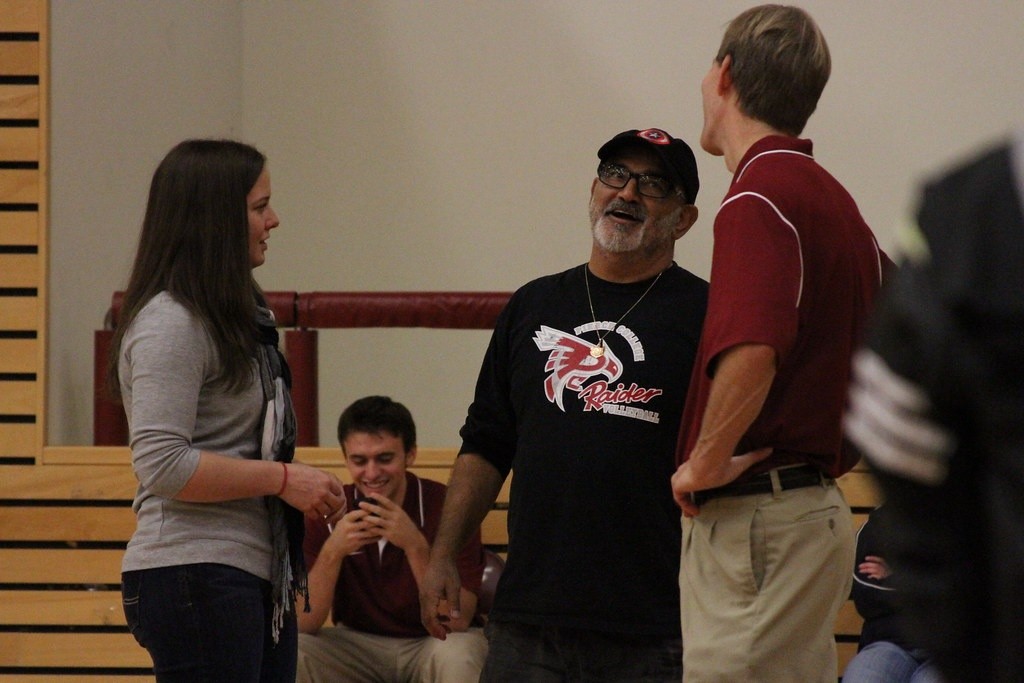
0,447 -> 885,683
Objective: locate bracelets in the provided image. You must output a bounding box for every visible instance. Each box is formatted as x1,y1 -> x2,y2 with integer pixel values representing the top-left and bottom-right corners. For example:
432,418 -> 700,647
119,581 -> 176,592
277,462 -> 289,496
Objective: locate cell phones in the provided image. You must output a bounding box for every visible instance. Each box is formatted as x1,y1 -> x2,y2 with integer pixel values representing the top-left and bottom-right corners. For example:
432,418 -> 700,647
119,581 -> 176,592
353,498 -> 383,537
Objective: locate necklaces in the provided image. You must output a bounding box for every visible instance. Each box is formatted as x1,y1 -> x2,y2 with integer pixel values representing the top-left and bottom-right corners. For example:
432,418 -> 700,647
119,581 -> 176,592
585,262 -> 666,358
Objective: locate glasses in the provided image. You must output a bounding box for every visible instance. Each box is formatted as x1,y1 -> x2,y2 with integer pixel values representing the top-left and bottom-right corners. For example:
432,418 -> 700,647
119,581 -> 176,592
597,160 -> 674,198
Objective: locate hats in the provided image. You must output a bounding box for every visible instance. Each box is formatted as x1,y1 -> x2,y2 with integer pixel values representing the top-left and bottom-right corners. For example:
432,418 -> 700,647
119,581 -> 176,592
597,128 -> 700,205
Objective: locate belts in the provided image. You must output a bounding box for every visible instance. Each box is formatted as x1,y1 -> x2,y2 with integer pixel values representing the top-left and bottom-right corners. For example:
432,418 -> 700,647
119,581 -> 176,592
683,451 -> 863,507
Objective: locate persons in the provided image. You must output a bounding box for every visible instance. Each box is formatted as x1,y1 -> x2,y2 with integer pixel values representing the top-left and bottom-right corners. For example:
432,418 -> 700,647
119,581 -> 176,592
419,127 -> 711,683
294,396 -> 488,683
674,4 -> 1024,683
106,138 -> 348,683
